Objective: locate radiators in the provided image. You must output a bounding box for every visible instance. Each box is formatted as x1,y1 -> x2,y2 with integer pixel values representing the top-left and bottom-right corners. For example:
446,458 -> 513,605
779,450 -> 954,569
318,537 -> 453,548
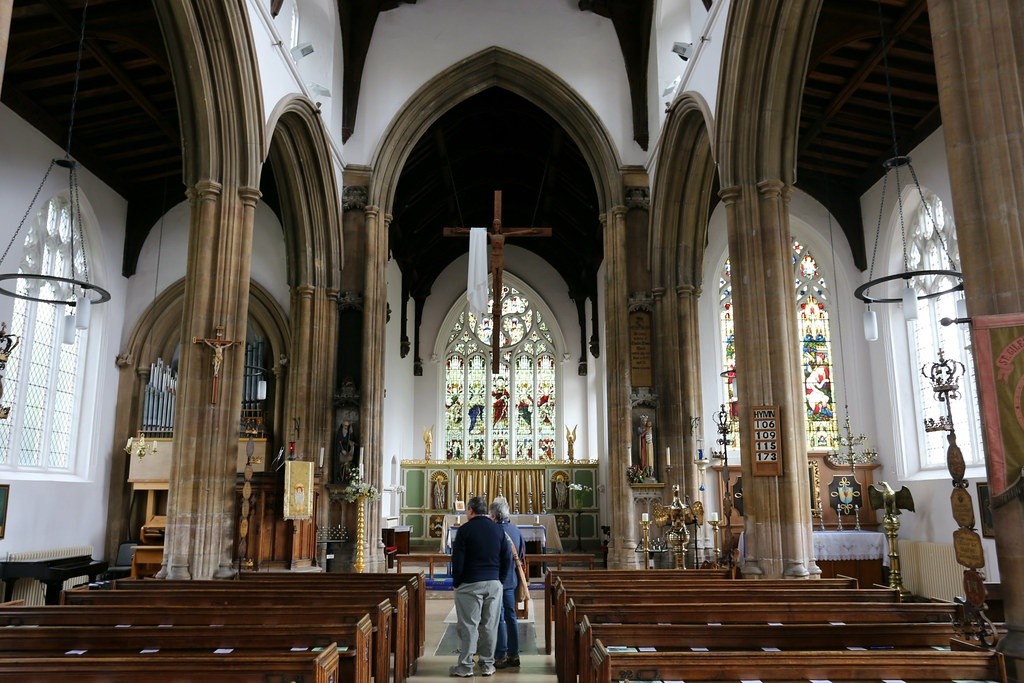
897,539 -> 971,604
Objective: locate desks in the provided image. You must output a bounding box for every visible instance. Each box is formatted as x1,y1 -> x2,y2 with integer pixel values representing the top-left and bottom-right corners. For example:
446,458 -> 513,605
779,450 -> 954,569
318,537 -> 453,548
541,566 -> 1012,683
0,572 -> 427,683
446,525 -> 545,577
396,553 -> 595,580
739,529 -> 892,591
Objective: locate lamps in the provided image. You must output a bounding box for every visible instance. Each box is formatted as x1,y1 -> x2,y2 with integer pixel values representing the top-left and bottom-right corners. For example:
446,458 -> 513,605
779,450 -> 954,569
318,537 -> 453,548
241,365 -> 271,406
826,207 -> 880,475
0,0 -> 111,345
854,0 -> 965,342
123,432 -> 158,464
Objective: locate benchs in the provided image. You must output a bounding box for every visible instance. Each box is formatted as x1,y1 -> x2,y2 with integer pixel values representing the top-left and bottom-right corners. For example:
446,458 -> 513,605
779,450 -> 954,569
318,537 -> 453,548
73,580 -> 112,590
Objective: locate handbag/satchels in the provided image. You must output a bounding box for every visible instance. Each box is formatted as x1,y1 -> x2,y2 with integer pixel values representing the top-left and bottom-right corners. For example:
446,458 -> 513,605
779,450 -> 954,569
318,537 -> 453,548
516,564 -> 530,601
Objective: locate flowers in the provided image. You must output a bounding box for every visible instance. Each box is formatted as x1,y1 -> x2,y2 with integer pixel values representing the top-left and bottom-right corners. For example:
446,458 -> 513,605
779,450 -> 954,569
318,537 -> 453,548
625,464 -> 647,483
568,483 -> 592,494
342,481 -> 381,506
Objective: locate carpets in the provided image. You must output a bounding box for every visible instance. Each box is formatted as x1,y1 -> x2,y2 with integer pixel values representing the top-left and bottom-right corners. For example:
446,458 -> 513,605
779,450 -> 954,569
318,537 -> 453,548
442,598 -> 536,623
423,574 -> 546,590
433,623 -> 539,656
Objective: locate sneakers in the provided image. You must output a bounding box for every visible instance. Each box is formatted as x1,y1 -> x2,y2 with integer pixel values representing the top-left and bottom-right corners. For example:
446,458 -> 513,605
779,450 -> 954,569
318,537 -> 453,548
450,665 -> 473,677
481,663 -> 496,676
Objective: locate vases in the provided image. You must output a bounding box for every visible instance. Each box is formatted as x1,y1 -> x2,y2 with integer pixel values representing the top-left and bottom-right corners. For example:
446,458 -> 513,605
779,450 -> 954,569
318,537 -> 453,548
575,490 -> 582,509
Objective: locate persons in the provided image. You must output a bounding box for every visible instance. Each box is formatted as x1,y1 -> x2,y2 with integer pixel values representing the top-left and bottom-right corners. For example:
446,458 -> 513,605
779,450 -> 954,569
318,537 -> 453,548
488,497 -> 526,670
336,419 -> 356,483
448,497 -> 513,679
637,413 -> 654,477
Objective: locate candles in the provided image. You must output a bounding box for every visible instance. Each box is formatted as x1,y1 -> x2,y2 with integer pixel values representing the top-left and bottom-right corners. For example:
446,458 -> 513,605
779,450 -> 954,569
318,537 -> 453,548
359,447 -> 363,465
320,447 -> 324,467
455,475 -> 544,493
534,516 -> 539,524
819,502 -> 822,509
711,512 -> 718,521
855,505 -> 858,509
666,447 -> 670,466
628,447 -> 632,467
838,504 -> 841,509
642,512 -> 648,522
457,516 -> 461,525
649,443 -> 653,466
149,357 -> 177,394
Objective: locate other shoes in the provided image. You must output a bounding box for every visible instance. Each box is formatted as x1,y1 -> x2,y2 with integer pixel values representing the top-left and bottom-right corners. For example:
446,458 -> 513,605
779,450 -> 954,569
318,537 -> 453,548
504,656 -> 521,666
493,658 -> 506,669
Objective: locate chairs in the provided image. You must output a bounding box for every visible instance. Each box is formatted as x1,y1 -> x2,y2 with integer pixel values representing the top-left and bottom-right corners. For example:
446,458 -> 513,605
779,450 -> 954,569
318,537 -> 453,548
108,543 -> 138,581
